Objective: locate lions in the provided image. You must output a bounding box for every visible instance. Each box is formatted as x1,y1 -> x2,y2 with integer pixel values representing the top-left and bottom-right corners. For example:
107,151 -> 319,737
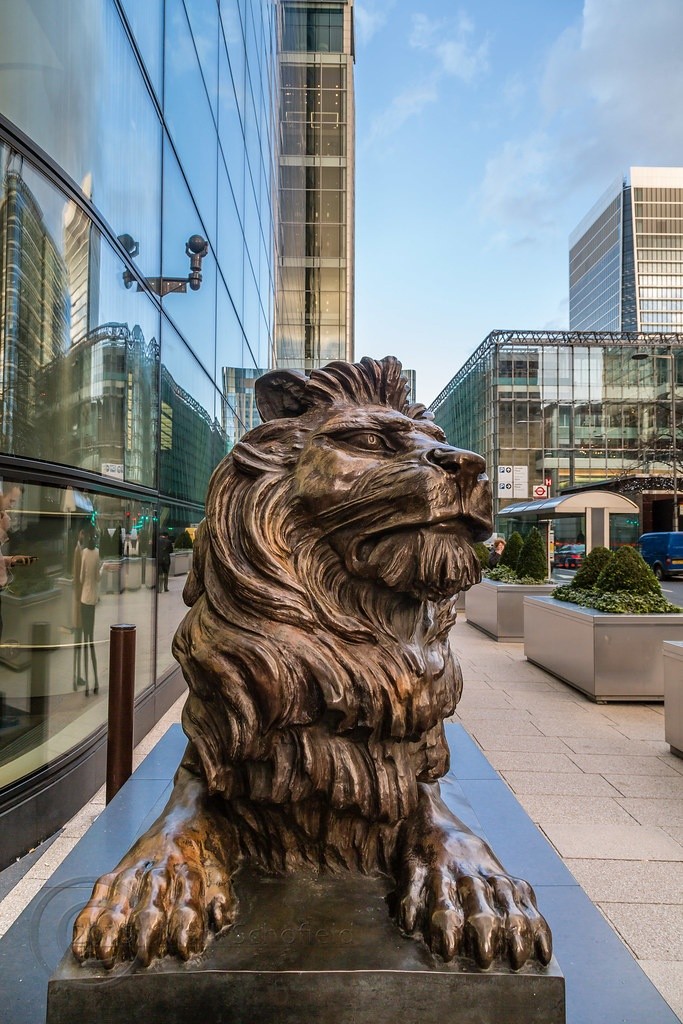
70,354 -> 553,973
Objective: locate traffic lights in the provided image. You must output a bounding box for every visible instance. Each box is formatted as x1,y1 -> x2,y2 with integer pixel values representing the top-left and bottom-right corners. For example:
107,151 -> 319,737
126,511 -> 158,524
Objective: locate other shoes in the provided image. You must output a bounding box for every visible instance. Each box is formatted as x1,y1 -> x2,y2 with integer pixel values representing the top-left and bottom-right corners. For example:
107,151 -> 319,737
73,682 -> 77,691
165,589 -> 169,592
77,678 -> 85,686
93,687 -> 98,695
85,690 -> 89,697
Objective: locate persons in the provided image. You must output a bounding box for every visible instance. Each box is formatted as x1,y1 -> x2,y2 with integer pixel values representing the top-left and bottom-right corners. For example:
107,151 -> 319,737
577,530 -> 584,544
80,527 -> 101,697
0,481 -> 39,728
489,538 -> 506,571
71,525 -> 96,691
156,532 -> 173,592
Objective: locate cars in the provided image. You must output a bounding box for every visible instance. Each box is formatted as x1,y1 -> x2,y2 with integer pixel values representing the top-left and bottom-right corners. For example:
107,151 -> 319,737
554,544 -> 586,568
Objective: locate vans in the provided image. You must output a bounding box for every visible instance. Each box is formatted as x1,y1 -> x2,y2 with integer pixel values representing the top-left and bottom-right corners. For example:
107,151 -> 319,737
638,531 -> 683,581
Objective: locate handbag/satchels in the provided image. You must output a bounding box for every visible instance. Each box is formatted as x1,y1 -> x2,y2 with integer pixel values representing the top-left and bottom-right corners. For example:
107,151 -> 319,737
159,550 -> 170,565
